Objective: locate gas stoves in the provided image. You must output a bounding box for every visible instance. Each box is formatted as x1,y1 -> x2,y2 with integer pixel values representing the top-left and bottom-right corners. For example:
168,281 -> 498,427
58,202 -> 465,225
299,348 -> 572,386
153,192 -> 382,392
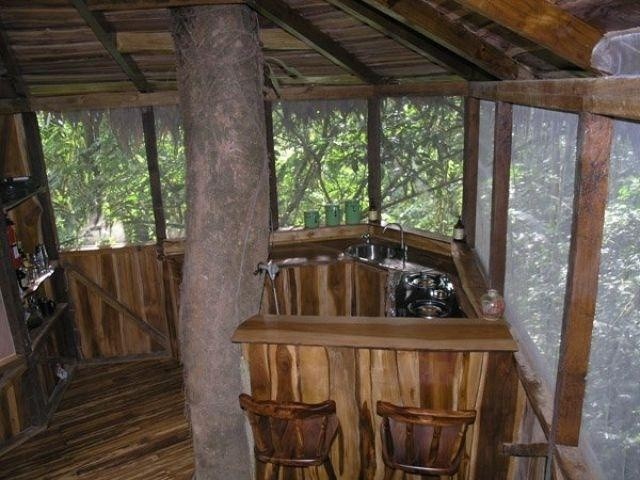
395,271 -> 468,319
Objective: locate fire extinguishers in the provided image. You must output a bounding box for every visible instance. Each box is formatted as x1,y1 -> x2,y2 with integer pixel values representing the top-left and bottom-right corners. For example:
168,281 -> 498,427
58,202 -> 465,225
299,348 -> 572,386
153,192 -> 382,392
5,216 -> 21,269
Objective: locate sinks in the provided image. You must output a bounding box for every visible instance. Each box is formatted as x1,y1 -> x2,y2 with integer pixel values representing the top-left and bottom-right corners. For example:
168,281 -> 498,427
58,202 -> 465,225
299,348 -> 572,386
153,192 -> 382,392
344,241 -> 397,267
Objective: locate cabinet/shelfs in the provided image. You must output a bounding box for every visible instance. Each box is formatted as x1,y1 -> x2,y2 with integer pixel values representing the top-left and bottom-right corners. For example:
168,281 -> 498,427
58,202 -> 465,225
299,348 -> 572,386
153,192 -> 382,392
356,260 -> 388,316
0,113 -> 81,414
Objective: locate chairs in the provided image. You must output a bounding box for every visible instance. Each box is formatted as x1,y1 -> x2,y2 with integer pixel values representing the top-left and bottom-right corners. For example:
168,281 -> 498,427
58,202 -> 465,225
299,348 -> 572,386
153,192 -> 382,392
238,394 -> 343,480
377,401 -> 477,480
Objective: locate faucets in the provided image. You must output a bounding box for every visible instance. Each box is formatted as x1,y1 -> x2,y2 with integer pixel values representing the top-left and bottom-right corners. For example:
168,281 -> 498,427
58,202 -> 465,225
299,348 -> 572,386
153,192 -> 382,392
381,221 -> 404,249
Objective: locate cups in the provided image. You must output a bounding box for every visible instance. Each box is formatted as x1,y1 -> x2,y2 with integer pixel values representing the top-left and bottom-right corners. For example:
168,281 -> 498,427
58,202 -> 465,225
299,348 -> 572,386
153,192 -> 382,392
304,199 -> 360,229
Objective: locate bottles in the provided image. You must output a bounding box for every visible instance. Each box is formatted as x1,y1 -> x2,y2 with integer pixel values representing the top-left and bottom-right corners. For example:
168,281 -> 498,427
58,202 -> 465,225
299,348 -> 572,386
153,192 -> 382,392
39,296 -> 56,317
479,289 -> 505,321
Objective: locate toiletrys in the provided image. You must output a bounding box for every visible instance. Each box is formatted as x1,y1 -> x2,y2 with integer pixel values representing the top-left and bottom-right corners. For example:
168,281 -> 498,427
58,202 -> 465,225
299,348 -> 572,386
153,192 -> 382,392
452,215 -> 465,241
